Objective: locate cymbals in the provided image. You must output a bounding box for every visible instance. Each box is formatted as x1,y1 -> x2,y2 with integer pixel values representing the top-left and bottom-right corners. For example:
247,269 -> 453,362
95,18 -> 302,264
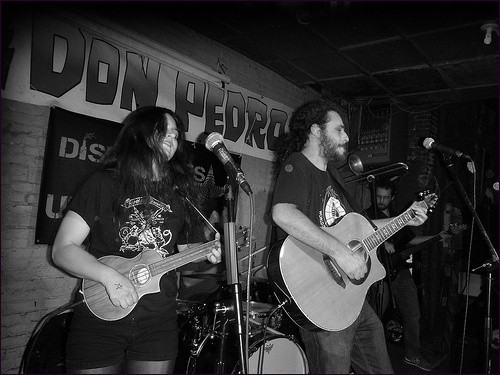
179,271 -> 268,283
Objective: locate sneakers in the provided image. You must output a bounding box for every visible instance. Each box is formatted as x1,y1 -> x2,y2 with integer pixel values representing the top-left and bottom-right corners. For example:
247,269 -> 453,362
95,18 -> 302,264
403,351 -> 433,371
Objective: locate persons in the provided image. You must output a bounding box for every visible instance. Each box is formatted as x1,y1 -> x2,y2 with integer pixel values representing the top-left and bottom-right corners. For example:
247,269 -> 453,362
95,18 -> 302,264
52,107 -> 225,374
365,181 -> 451,371
271,101 -> 428,374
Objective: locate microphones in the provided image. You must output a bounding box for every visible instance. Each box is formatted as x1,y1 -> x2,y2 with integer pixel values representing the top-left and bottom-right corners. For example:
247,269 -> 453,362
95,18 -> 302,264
423,137 -> 472,160
205,132 -> 254,196
367,174 -> 375,182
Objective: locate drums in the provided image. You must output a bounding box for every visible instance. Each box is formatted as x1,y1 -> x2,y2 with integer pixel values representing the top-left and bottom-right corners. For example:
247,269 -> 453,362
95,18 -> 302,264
185,321 -> 311,375
176,297 -> 283,375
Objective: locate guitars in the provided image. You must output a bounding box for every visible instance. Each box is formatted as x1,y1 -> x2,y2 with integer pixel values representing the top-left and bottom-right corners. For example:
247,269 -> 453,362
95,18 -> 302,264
379,221 -> 469,283
266,188 -> 441,333
80,226 -> 254,321
